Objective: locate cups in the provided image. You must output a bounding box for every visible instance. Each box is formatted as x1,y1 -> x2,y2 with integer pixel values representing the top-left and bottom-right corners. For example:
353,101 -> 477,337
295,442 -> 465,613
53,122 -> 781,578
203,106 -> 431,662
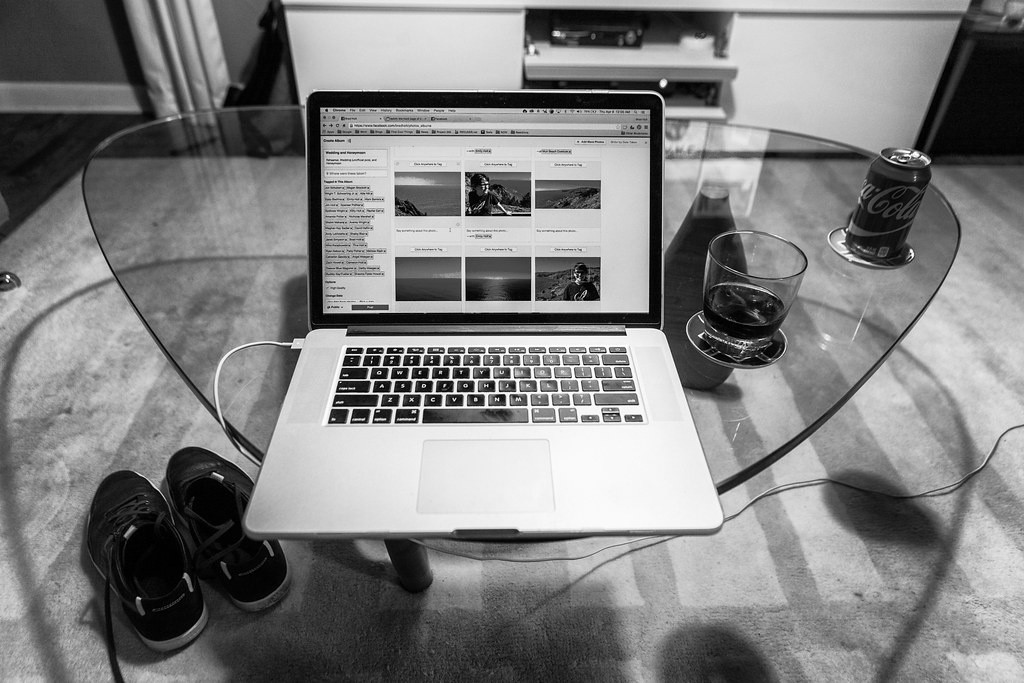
702,229 -> 808,359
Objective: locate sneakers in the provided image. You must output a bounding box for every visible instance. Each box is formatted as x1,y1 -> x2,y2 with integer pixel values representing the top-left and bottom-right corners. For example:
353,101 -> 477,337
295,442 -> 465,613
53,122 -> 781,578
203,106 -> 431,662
86,468 -> 209,683
164,445 -> 291,611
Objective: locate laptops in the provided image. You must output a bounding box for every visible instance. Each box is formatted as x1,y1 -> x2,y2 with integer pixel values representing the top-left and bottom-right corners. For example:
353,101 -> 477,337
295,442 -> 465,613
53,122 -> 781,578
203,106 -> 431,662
243,89 -> 724,540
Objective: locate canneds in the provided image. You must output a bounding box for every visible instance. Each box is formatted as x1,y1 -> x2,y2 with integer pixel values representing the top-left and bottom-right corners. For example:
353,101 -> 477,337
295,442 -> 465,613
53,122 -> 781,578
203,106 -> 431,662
846,147 -> 931,260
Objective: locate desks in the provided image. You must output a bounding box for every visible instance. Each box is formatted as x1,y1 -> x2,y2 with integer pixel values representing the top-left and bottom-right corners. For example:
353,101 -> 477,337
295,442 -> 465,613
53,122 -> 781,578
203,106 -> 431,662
81,105 -> 962,594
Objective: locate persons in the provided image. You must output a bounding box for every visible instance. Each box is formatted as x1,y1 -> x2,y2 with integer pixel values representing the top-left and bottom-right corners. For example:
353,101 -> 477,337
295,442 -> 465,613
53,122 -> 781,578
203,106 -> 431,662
463,172 -> 512,216
564,264 -> 598,300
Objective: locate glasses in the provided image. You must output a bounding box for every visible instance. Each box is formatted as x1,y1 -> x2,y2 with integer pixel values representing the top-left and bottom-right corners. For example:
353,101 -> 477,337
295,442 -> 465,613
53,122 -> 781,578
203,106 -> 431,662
575,271 -> 586,275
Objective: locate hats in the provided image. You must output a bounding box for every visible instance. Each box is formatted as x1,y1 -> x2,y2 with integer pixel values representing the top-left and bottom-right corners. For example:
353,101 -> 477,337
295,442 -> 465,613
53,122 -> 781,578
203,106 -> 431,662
573,262 -> 587,273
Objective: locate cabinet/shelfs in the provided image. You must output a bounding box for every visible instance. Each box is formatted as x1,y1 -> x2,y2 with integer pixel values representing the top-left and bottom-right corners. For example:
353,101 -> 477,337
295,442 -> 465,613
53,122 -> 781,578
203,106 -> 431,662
523,41 -> 740,124
